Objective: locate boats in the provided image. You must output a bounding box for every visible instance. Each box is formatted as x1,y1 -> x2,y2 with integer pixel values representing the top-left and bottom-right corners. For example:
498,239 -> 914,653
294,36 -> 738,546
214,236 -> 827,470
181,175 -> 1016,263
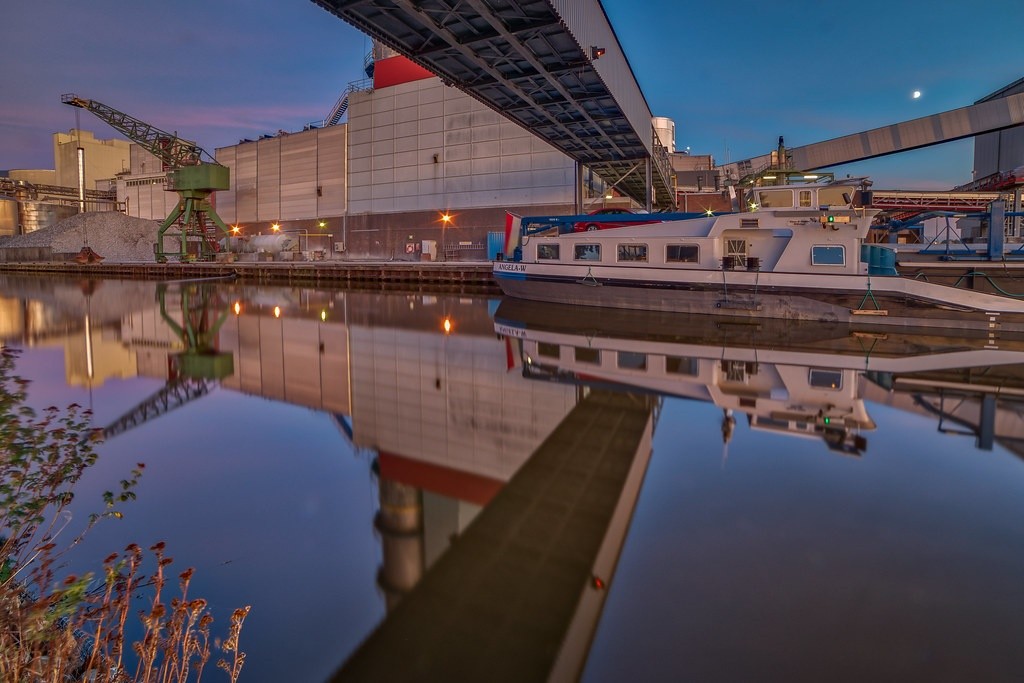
493,295 -> 1024,459
492,191 -> 1023,312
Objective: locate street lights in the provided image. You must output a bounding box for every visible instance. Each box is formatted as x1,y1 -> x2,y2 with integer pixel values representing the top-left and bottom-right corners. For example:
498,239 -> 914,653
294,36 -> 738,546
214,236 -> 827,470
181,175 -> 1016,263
442,216 -> 450,252
